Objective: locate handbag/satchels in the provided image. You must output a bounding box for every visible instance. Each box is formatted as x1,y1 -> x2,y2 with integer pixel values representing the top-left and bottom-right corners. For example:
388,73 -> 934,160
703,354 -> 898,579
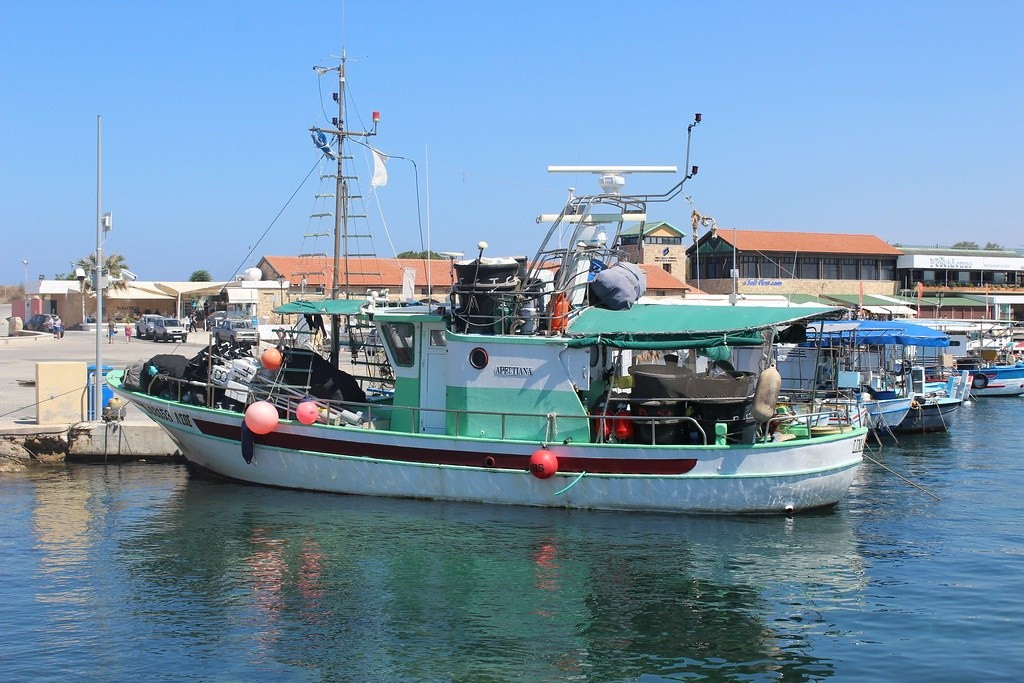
114,331 -> 118,334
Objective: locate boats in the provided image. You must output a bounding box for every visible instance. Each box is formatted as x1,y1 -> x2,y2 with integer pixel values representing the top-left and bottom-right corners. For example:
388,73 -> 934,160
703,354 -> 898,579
102,51 -> 1024,515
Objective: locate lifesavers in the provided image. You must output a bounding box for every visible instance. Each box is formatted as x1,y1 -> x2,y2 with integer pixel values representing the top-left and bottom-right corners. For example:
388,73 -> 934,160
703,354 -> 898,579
973,373 -> 989,389
901,361 -> 912,375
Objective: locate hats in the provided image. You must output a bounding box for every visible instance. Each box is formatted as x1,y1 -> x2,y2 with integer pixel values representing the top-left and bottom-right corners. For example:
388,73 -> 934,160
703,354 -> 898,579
192,312 -> 196,315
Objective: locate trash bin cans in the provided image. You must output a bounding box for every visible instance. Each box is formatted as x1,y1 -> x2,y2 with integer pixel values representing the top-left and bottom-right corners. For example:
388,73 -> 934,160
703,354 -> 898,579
87,365 -> 114,421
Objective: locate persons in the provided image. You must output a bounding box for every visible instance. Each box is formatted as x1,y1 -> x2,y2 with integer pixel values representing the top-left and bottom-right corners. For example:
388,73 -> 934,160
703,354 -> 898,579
125,321 -> 132,343
204,300 -> 210,320
981,314 -> 986,319
143,306 -> 152,314
189,310 -> 198,332
43,314 -> 61,340
851,310 -> 887,321
154,308 -> 162,316
107,319 -> 114,343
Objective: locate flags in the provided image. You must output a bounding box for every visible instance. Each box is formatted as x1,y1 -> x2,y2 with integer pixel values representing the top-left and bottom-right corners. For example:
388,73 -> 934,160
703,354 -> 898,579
313,131 -> 336,161
370,146 -> 390,186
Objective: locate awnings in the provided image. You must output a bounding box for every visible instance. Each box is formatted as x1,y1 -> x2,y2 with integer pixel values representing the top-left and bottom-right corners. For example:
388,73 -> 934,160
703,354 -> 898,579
782,294 -> 845,306
819,293 -> 994,307
859,306 -> 917,315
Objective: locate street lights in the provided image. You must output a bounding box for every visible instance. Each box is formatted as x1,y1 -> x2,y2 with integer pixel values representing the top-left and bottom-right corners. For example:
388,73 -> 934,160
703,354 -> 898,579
690,211 -> 718,289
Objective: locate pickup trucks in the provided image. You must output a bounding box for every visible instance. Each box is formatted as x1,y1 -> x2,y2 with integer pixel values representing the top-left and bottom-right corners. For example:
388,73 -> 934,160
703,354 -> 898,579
211,320 -> 258,347
134,314 -> 164,340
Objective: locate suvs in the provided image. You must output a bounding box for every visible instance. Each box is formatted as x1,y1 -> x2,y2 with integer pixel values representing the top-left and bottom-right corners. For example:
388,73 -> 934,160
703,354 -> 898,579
24,314 -> 62,333
153,317 -> 188,343
